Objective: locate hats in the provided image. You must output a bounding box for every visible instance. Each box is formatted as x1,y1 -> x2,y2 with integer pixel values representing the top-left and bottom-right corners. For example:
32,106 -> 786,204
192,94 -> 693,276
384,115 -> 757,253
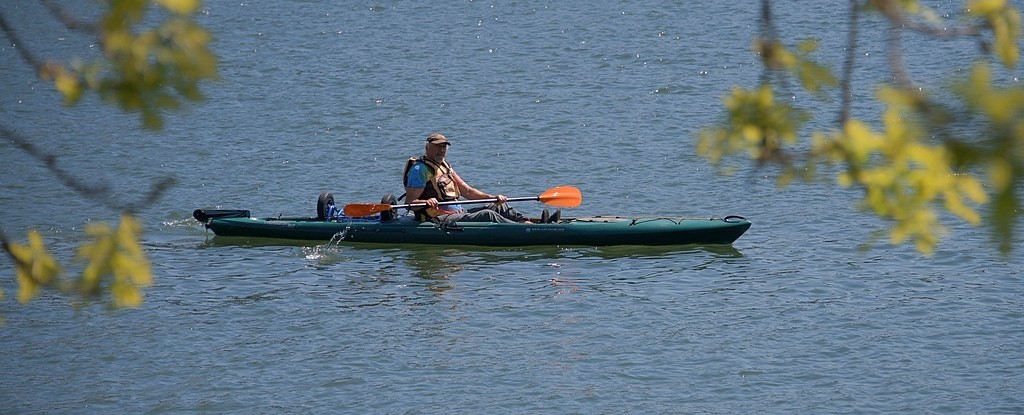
426,134 -> 451,146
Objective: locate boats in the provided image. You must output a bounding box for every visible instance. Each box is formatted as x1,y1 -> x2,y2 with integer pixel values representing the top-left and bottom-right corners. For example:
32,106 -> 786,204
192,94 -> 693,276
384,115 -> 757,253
192,207 -> 754,248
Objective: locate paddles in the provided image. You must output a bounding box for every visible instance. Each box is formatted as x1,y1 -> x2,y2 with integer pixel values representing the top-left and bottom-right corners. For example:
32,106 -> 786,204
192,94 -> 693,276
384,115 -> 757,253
345,184 -> 583,218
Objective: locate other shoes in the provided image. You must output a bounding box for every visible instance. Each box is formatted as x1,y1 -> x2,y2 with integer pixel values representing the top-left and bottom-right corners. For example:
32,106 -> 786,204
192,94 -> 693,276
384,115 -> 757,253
538,208 -> 549,223
549,209 -> 561,223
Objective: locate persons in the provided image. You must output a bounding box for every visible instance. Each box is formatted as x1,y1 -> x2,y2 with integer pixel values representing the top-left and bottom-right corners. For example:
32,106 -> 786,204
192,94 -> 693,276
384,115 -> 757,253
404,133 -> 561,224
